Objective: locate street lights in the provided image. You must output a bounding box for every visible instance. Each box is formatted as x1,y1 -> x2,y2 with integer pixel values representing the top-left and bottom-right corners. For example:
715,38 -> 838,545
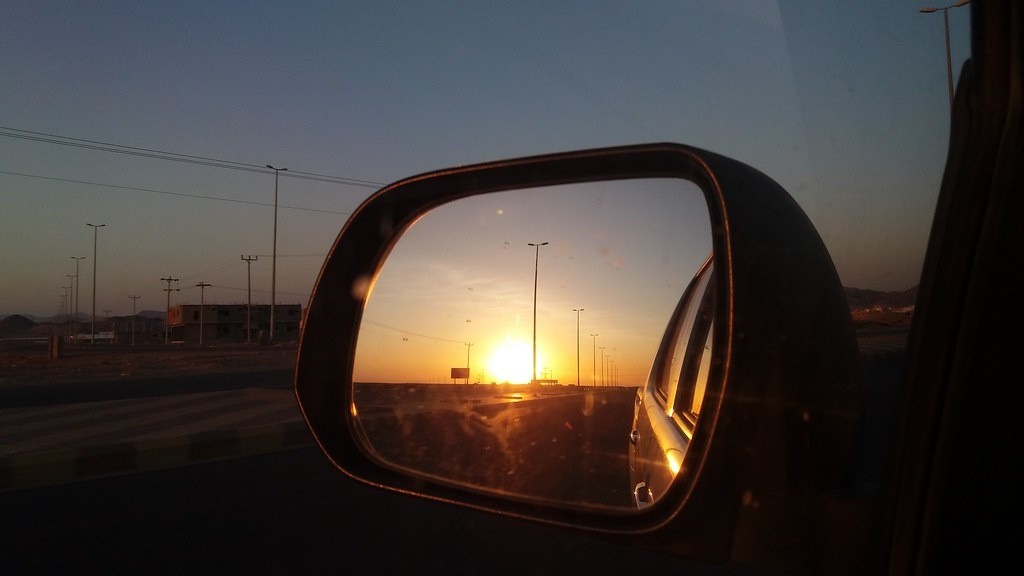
590,333 -> 599,386
527,242 -> 550,397
919,1 -> 970,136
598,346 -> 620,390
572,308 -> 585,386
59,223 -> 106,345
265,164 -> 288,340
